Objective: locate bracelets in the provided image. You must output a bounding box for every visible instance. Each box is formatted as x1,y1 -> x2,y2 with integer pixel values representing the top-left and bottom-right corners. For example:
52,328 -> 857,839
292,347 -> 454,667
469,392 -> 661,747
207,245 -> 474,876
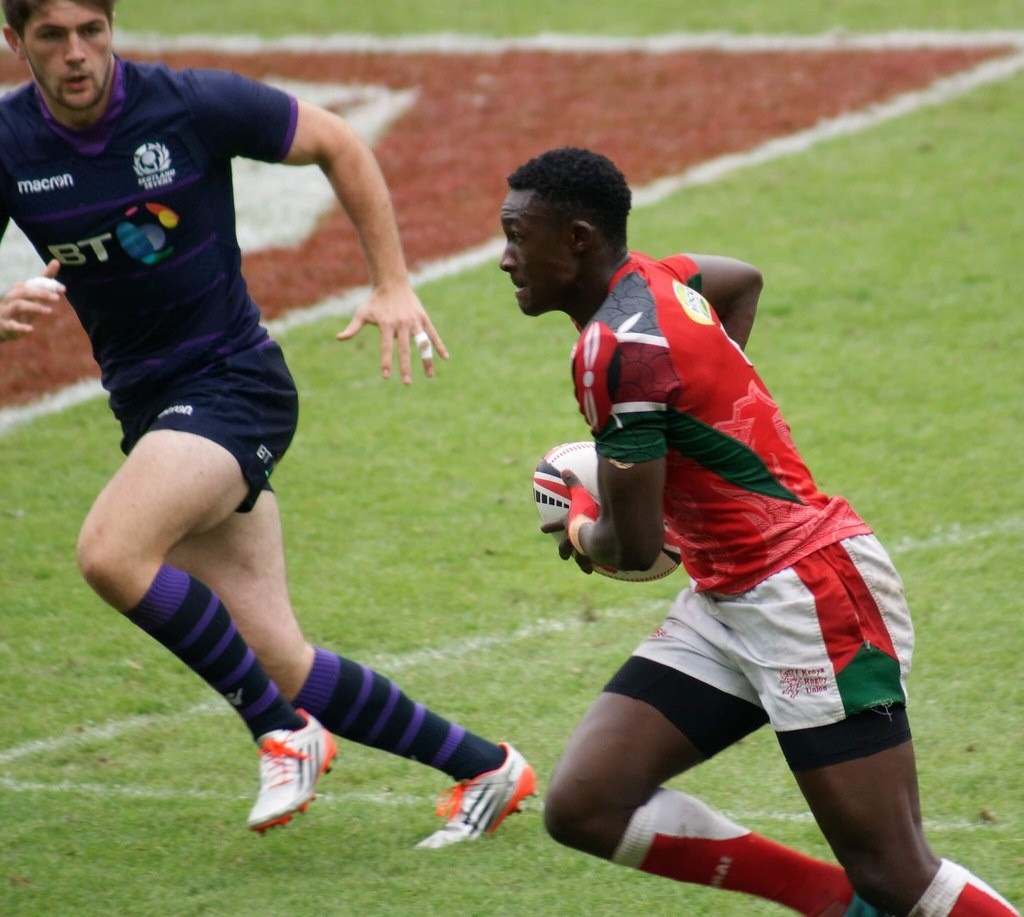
567,514 -> 594,556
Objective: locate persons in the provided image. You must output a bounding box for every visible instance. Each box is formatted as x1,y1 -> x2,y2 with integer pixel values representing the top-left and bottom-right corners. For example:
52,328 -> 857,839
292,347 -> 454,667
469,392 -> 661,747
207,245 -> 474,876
497,142 -> 1024,916
1,0 -> 538,851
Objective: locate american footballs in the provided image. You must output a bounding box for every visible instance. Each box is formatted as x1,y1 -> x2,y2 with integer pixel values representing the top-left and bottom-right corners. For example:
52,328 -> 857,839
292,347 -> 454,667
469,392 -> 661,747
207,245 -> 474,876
532,441 -> 683,583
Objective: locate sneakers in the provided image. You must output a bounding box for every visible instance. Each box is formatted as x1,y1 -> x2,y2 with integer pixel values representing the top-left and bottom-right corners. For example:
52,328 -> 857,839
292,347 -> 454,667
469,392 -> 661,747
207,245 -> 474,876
414,743 -> 537,848
245,707 -> 337,837
